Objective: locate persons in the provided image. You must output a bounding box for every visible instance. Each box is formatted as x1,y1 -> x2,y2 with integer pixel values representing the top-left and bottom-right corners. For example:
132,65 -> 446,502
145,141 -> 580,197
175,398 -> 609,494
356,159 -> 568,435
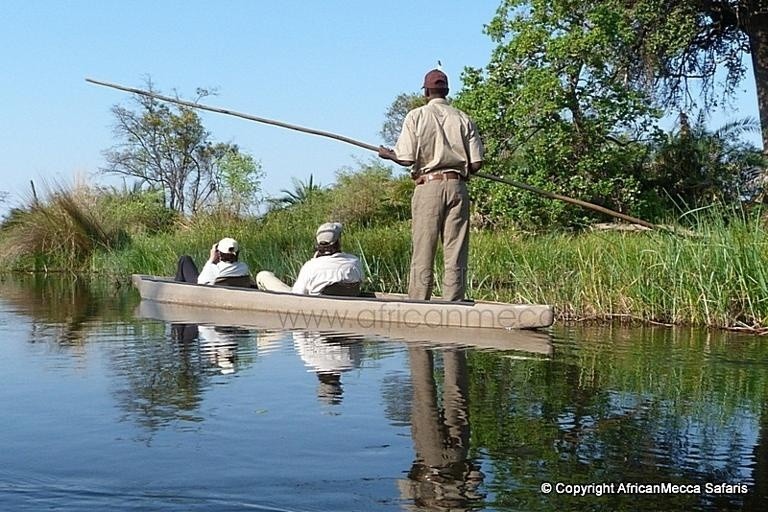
175,237 -> 249,285
256,328 -> 365,406
394,342 -> 485,512
254,220 -> 365,295
170,324 -> 251,375
378,68 -> 486,300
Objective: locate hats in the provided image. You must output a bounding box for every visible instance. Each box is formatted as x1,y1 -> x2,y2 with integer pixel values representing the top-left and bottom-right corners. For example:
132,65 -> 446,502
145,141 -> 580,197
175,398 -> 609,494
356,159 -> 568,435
316,222 -> 343,246
216,237 -> 238,256
421,70 -> 448,89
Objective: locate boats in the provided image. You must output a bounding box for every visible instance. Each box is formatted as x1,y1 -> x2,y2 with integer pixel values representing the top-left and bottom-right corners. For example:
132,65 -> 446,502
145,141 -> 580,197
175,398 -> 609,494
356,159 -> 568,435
128,272 -> 556,331
137,298 -> 557,363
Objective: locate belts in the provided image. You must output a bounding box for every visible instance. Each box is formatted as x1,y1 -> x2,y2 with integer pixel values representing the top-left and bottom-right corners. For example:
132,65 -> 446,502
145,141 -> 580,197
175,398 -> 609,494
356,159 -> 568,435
415,174 -> 458,184
